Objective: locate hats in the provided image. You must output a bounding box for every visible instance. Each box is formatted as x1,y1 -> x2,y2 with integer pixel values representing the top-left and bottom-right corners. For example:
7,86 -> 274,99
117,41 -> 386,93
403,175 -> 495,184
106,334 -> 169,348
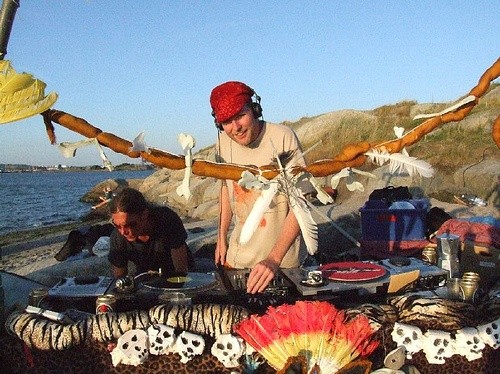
210,80 -> 255,123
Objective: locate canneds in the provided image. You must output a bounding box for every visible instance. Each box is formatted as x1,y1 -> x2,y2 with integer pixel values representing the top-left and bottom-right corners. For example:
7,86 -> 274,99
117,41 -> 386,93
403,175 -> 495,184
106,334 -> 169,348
30,288 -> 49,309
421,247 -> 436,265
446,278 -> 461,301
460,272 -> 480,302
95,293 -> 118,315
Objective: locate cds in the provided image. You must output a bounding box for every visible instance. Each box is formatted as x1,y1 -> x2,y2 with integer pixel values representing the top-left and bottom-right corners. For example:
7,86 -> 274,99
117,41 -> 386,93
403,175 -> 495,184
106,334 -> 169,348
146,273 -> 216,291
320,262 -> 388,282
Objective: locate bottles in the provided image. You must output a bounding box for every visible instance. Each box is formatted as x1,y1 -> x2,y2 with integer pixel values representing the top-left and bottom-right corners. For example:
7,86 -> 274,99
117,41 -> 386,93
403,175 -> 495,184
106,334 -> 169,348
437,228 -> 461,278
115,277 -> 133,289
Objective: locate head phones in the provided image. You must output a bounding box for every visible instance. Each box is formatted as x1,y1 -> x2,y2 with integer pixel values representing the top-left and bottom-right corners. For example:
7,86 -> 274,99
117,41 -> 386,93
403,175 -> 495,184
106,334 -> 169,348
210,86 -> 263,132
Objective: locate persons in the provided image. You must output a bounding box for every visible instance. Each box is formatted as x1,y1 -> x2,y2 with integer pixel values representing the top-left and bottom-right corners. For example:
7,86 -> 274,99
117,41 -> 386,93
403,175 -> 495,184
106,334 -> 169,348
107,188 -> 196,280
208,81 -> 308,295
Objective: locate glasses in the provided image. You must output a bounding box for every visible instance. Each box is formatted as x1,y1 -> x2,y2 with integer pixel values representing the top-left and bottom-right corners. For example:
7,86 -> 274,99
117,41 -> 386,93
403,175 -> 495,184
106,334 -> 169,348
113,221 -> 138,229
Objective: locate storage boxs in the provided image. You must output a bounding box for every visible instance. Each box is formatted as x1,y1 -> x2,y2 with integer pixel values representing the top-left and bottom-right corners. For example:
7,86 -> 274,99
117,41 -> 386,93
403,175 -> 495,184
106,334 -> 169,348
458,239 -> 500,289
358,198 -> 431,251
